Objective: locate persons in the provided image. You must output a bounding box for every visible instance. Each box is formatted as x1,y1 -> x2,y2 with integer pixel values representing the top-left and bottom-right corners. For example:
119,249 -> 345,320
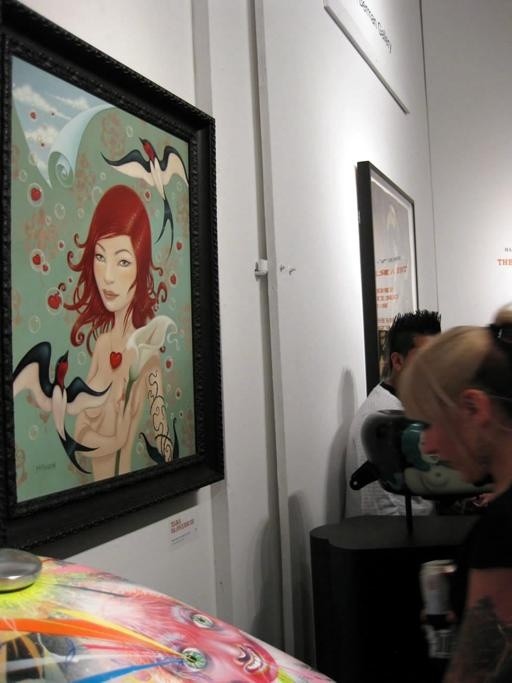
397,301 -> 511,680
59,182 -> 175,484
341,307 -> 445,515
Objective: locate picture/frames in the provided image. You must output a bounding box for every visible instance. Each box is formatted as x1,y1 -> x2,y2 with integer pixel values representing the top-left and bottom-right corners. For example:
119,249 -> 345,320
358,161 -> 419,400
0,0 -> 225,549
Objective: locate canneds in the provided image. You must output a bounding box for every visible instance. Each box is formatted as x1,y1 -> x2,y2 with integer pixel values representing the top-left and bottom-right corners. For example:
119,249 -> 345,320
422,560 -> 457,614
